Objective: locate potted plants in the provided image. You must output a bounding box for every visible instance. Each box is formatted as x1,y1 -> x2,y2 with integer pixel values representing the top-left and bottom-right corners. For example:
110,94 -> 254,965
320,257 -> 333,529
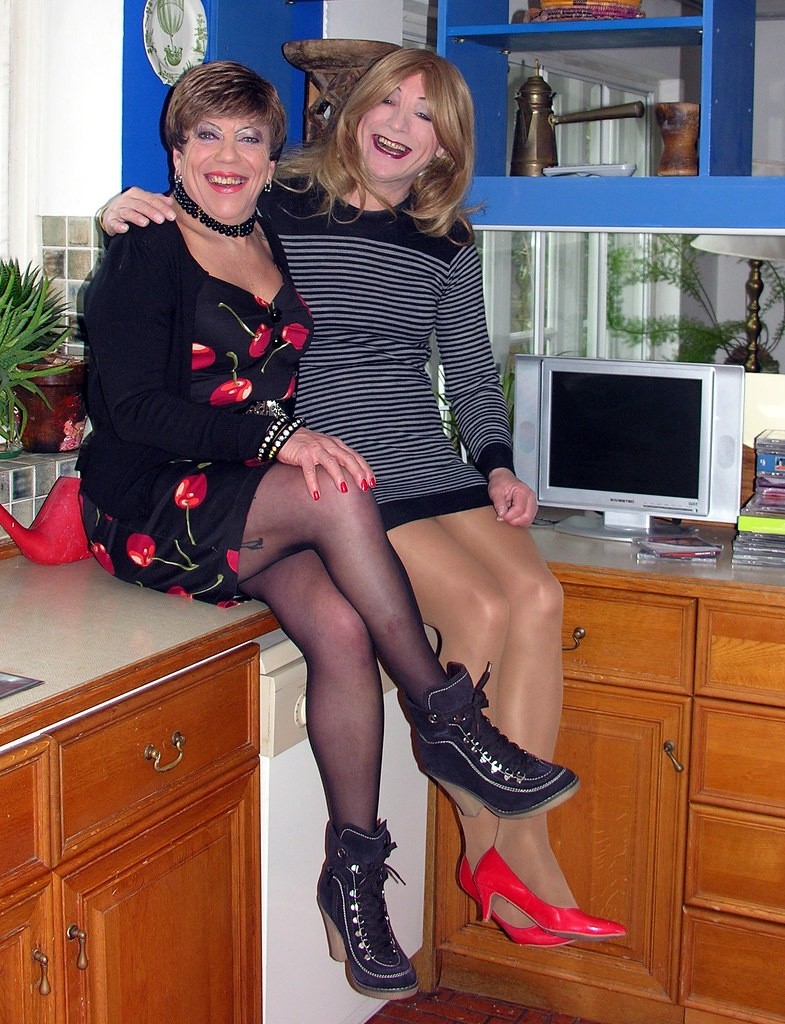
0,259 -> 91,453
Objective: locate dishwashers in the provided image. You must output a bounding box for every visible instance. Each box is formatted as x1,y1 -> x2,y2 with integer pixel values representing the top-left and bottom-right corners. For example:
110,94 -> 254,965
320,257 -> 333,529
259,638 -> 429,1024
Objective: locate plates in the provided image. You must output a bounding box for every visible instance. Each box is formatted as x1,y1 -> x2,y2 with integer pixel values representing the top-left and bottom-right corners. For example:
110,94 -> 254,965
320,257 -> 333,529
143,0 -> 208,87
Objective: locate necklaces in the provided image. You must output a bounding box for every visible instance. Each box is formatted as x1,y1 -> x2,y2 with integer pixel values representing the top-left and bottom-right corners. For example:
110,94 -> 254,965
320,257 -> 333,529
172,183 -> 256,236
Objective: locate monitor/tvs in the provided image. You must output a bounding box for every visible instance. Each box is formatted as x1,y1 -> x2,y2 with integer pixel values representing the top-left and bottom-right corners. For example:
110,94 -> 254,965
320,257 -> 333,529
512,354 -> 745,542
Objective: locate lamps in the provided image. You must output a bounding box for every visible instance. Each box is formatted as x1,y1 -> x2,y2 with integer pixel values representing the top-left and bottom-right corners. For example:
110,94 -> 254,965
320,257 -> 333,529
688,233 -> 785,372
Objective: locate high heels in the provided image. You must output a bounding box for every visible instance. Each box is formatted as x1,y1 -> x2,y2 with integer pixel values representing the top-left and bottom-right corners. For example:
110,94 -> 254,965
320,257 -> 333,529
316,818 -> 418,1000
401,660 -> 581,821
459,855 -> 578,948
472,846 -> 628,940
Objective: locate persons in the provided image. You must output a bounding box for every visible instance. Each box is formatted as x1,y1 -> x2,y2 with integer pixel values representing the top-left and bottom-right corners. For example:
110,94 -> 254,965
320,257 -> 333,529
94,50 -> 629,948
80,59 -> 580,1000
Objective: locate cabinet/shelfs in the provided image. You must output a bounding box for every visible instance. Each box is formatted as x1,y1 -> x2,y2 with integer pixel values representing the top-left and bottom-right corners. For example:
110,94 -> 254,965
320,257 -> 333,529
0,563 -> 784,1024
438,0 -> 785,231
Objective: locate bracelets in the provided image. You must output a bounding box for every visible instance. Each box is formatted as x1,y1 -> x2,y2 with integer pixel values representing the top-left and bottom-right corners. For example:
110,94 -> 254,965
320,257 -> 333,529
258,418 -> 304,463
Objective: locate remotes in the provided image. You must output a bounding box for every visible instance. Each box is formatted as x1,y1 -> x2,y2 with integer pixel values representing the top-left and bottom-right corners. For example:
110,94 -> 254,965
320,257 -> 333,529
543,162 -> 637,178
